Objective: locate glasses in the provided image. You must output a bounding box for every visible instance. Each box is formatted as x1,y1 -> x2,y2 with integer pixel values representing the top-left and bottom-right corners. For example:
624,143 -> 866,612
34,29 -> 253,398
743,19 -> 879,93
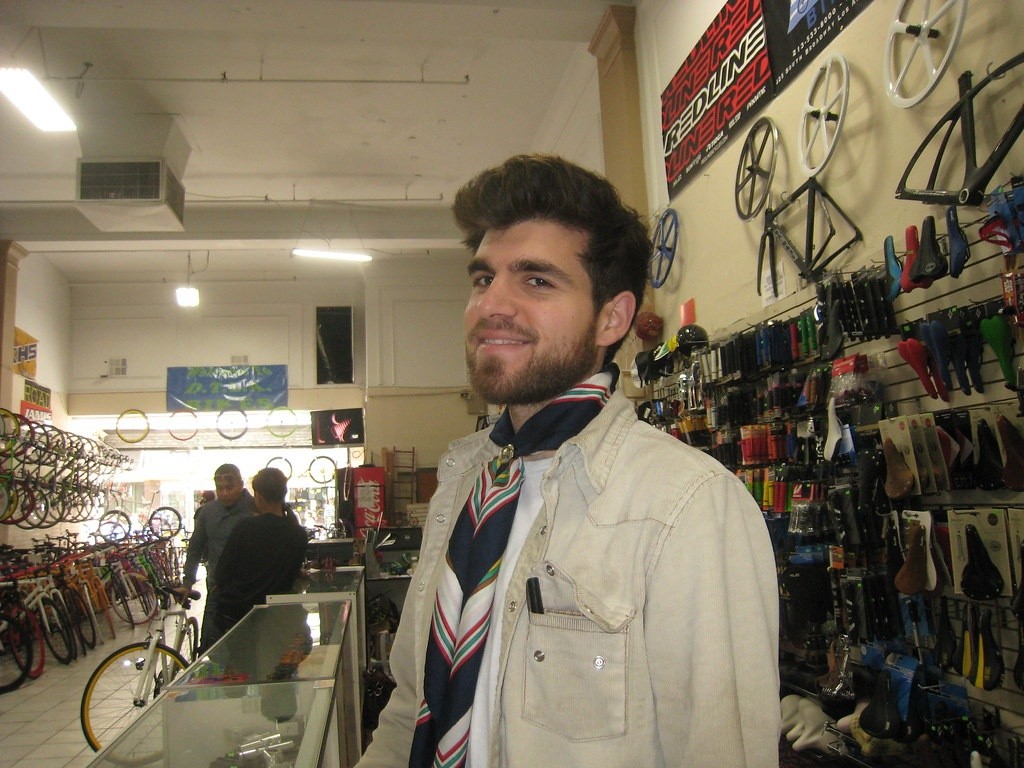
214,475 -> 240,483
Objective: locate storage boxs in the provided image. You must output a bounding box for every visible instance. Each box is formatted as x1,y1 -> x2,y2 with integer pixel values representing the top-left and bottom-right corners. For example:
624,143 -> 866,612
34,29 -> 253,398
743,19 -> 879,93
308,539 -> 354,561
375,528 -> 422,552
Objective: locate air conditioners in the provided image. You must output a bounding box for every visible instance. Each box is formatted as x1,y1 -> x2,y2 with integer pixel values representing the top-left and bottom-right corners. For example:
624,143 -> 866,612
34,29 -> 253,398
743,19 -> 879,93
72,157 -> 186,232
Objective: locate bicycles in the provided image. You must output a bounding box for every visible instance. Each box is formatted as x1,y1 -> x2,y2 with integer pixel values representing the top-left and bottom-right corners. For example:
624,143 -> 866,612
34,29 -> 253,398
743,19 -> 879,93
0,523 -> 196,696
80,586 -> 199,768
0,408 -> 136,530
98,490 -> 182,543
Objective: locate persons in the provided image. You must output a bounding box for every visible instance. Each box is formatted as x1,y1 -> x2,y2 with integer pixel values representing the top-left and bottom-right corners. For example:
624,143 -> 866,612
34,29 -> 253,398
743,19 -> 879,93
212,605 -> 314,722
182,464 -> 256,658
353,153 -> 782,767
194,490 -> 216,531
213,467 -> 308,637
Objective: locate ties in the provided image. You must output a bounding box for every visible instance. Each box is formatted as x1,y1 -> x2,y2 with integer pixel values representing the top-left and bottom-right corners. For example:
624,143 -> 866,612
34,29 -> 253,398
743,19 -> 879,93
408,359 -> 620,768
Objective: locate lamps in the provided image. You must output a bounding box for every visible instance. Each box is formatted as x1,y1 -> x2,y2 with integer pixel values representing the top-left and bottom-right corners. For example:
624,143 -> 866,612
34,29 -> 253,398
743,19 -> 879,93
177,251 -> 201,309
0,25 -> 96,134
291,199 -> 373,263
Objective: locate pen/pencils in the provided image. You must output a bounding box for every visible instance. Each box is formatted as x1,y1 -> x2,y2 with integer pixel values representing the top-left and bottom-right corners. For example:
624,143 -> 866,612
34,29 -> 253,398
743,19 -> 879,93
524,575 -> 544,614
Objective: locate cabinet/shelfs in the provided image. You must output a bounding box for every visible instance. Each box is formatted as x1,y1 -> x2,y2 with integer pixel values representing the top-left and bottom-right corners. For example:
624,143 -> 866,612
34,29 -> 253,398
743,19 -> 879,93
86,599 -> 357,768
265,564 -> 366,760
